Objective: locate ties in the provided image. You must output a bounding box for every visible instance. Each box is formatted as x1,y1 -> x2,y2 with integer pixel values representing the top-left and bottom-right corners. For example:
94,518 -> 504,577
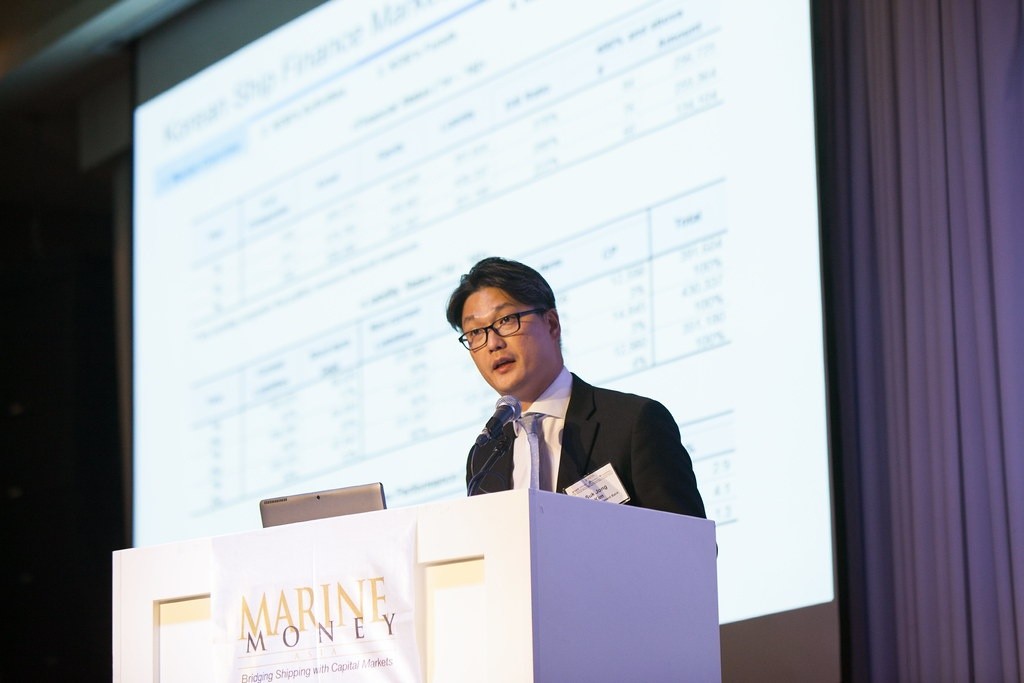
515,413 -> 547,491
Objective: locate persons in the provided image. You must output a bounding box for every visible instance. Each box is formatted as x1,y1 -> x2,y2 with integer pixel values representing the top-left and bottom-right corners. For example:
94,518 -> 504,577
445,256 -> 718,560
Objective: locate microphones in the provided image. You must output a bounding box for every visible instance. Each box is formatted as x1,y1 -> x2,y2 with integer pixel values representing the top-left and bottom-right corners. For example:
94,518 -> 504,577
475,395 -> 522,447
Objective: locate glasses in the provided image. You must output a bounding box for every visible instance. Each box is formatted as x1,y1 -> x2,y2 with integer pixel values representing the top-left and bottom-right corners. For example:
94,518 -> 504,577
458,309 -> 545,351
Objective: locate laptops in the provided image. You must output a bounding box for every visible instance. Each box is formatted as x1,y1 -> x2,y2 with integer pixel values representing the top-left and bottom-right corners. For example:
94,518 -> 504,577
260,482 -> 388,528
